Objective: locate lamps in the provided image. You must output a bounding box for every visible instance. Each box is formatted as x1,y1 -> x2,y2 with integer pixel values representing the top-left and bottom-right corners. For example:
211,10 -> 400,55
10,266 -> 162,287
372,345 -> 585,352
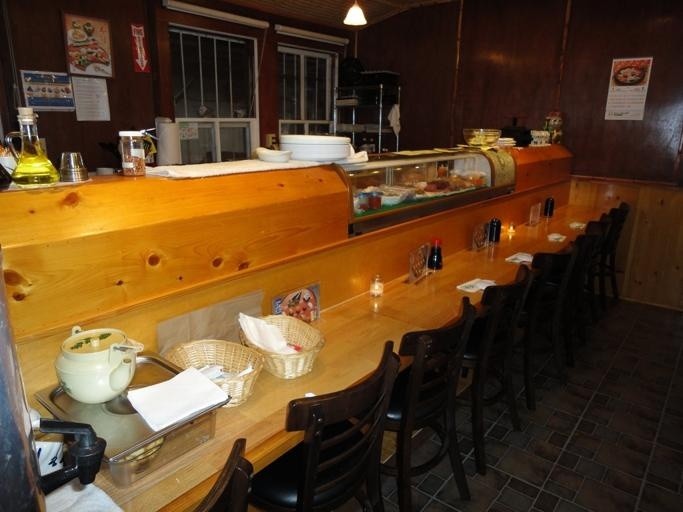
343,0 -> 367,25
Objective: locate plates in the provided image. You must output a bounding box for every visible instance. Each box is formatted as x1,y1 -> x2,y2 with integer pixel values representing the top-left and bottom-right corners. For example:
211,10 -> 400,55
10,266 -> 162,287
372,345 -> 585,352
493,136 -> 515,148
255,134 -> 352,162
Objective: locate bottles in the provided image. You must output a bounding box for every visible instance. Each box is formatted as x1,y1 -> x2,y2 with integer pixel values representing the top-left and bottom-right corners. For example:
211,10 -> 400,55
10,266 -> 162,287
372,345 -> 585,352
357,191 -> 381,211
5,106 -> 60,190
544,195 -> 554,218
490,217 -> 500,244
359,137 -> 376,154
118,129 -> 147,176
368,273 -> 384,298
428,236 -> 443,272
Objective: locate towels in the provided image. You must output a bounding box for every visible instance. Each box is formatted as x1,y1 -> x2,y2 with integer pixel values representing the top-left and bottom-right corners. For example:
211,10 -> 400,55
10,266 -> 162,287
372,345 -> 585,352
127,367 -> 230,433
387,104 -> 401,136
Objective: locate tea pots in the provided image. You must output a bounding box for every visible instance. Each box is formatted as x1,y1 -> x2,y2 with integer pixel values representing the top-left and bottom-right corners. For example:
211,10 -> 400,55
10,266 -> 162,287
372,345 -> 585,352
53,324 -> 144,406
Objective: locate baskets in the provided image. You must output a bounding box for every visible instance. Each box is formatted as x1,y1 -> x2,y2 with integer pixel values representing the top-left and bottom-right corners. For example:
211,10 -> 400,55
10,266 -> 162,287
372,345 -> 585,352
238,314 -> 326,379
165,338 -> 264,409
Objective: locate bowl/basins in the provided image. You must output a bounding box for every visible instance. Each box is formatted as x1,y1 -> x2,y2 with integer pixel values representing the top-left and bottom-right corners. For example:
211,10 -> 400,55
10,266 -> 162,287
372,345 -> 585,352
460,126 -> 502,148
613,66 -> 645,86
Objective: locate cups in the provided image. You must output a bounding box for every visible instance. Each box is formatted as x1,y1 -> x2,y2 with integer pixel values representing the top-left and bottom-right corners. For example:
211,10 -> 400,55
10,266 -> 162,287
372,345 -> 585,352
528,130 -> 551,145
59,150 -> 88,183
